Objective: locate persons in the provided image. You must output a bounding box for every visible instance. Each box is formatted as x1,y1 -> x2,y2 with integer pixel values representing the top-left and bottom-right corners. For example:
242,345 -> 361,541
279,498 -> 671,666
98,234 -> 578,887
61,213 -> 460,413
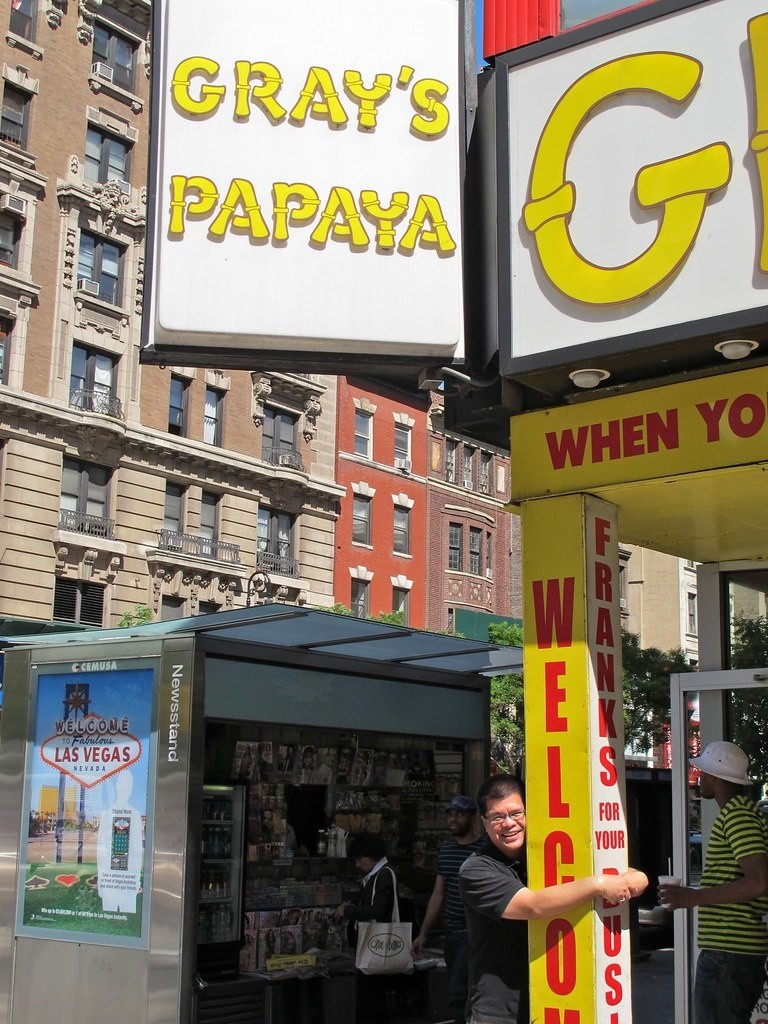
412,795 -> 490,1024
459,773 -> 650,1024
657,742 -> 768,1024
334,831 -> 404,1024
435,776 -> 462,802
300,747 -> 331,783
264,930 -> 276,959
281,933 -> 296,955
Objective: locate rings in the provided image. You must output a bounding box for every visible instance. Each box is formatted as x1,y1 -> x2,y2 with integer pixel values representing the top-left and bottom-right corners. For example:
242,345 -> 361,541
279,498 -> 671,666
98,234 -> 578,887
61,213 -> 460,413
618,897 -> 626,903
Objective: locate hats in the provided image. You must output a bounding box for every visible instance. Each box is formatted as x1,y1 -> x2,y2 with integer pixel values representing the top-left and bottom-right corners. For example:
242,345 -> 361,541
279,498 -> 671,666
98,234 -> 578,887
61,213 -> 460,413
688,741 -> 754,786
443,796 -> 477,815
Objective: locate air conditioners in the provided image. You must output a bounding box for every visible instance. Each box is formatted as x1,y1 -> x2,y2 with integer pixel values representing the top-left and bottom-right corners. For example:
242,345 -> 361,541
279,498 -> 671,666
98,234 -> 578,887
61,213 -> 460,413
395,459 -> 411,470
620,598 -> 626,608
486,568 -> 492,580
462,480 -> 473,490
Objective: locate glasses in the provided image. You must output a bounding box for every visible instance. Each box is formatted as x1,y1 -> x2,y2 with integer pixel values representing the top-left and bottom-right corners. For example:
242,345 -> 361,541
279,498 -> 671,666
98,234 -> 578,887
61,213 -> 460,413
483,809 -> 526,826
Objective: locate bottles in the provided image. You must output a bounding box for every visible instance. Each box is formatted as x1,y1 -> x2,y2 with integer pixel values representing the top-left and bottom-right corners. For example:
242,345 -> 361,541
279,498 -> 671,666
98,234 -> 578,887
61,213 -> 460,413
198,827 -> 231,857
194,905 -> 232,940
327,824 -> 337,857
315,829 -> 326,854
201,799 -> 231,820
197,866 -> 233,898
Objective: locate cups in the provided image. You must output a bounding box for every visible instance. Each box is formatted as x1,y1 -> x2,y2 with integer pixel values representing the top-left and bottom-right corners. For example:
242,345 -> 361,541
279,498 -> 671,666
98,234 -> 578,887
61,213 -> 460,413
657,875 -> 681,909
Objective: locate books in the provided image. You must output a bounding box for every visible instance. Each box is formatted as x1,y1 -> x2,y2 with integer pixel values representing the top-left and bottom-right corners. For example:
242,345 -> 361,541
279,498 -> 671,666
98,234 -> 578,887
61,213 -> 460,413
232,741 -> 469,977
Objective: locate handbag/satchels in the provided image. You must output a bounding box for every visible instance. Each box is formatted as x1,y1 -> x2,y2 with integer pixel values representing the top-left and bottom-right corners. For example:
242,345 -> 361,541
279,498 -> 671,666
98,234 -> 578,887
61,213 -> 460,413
355,867 -> 415,977
